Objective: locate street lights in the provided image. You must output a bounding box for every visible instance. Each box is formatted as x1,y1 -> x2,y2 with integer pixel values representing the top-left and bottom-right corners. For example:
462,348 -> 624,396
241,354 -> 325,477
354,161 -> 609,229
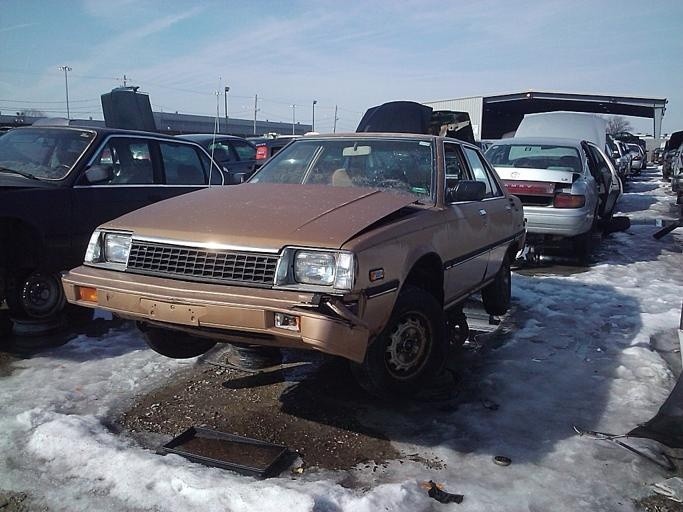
56,64 -> 73,118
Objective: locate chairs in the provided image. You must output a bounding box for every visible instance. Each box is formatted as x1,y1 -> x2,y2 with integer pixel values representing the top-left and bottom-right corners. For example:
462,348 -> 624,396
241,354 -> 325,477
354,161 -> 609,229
332,167 -> 363,188
